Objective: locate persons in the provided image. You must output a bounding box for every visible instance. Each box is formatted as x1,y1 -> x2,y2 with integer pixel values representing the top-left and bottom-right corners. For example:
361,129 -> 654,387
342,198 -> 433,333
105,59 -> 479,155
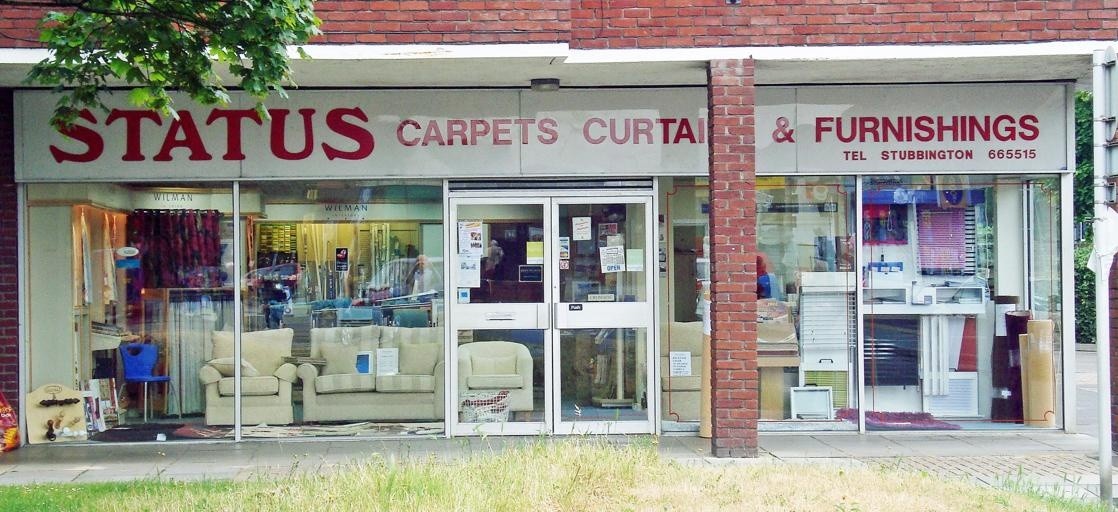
260,273 -> 274,329
405,253 -> 443,300
264,282 -> 289,330
484,237 -> 505,296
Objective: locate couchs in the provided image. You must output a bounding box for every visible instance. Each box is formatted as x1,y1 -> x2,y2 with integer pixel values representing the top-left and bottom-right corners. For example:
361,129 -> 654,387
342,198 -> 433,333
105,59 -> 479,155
197,327 -> 298,426
653,322 -> 706,423
454,339 -> 534,420
298,325 -> 451,422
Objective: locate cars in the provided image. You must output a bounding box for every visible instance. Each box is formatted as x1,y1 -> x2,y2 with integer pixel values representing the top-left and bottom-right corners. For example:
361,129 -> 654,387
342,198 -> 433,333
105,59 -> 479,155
241,264 -> 315,311
340,257 -> 444,347
946,273 -> 1061,328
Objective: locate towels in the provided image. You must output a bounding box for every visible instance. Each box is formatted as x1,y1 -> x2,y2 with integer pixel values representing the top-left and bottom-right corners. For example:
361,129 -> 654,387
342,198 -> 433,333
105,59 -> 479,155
104,215 -> 118,307
80,213 -> 94,307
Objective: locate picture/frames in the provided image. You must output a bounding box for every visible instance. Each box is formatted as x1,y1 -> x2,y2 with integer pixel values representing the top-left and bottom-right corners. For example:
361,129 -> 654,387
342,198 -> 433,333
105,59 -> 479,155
571,281 -> 601,302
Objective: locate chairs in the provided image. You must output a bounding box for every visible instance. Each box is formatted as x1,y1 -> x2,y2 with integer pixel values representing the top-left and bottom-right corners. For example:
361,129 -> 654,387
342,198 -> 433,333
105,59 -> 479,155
115,340 -> 182,425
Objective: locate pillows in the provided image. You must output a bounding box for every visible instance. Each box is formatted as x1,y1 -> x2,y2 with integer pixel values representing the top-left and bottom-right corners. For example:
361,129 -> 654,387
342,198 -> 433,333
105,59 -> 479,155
321,342 -> 359,374
207,357 -> 259,380
398,342 -> 437,376
472,354 -> 516,376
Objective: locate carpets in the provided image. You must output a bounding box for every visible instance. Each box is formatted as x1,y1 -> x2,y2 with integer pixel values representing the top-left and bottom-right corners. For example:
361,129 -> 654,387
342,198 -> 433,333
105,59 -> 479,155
836,406 -> 960,433
86,423 -> 206,439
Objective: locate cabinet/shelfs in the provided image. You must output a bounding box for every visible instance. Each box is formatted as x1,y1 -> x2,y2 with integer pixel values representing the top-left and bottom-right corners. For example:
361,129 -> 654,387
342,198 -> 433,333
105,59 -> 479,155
799,270 -> 858,419
852,175 -> 990,419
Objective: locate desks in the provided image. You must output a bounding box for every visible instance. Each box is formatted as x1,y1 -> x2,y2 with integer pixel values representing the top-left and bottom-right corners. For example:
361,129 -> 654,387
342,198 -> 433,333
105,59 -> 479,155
753,355 -> 801,420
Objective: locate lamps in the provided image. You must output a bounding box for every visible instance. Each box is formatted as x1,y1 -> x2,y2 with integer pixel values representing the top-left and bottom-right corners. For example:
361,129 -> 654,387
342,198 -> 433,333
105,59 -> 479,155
530,77 -> 560,92
